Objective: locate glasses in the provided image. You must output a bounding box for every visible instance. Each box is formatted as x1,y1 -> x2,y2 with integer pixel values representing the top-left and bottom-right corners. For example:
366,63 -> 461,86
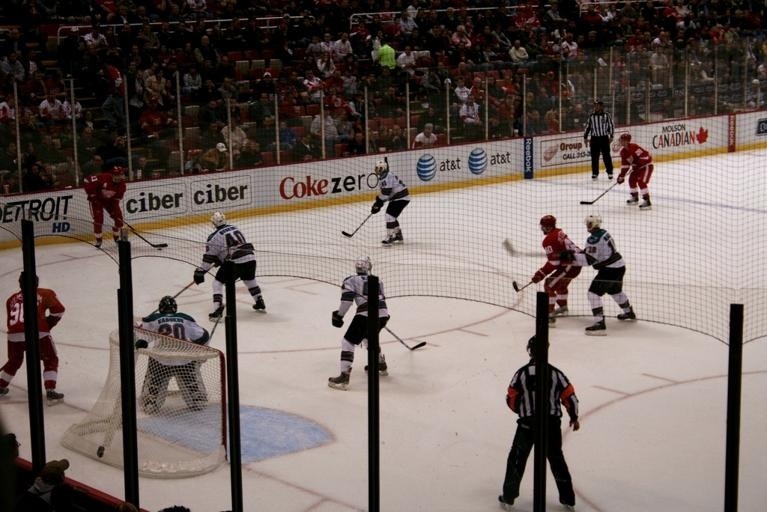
149,101 -> 156,105
15,441 -> 21,449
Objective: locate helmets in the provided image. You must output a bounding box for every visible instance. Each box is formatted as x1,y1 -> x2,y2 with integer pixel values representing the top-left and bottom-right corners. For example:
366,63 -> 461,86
584,214 -> 602,232
160,296 -> 177,313
527,335 -> 549,358
540,215 -> 555,235
593,99 -> 604,112
619,134 -> 631,146
211,211 -> 226,228
355,255 -> 371,273
110,166 -> 124,183
375,160 -> 388,174
19,272 -> 38,288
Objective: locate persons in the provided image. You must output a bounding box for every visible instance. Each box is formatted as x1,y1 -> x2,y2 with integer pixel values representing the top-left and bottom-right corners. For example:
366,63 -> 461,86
83,0 -> 129,178
328,254 -> 389,390
371,160 -> 410,246
532,215 -> 582,326
568,212 -> 636,336
83,165 -> 127,248
0,0 -> 83,193
49,484 -> 86,512
616,0 -> 767,126
499,333 -> 581,511
194,212 -> 266,319
135,296 -> 210,414
0,271 -> 66,401
616,133 -> 653,210
582,0 -> 616,101
129,154 -> 318,181
0,433 -> 28,512
26,459 -> 69,503
583,101 -> 615,179
128,0 -> 583,153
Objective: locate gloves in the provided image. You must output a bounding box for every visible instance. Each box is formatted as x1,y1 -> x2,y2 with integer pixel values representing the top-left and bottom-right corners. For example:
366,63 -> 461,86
560,250 -> 575,261
331,311 -> 343,328
371,196 -> 383,213
194,270 -> 204,284
532,269 -> 546,283
108,200 -> 120,218
89,193 -> 101,203
617,175 -> 624,184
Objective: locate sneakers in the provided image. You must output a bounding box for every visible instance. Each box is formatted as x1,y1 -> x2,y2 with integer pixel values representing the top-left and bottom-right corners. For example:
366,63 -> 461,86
609,174 -> 613,180
558,495 -> 575,506
627,196 -> 638,202
365,361 -> 386,371
549,316 -> 556,324
253,301 -> 266,309
328,373 -> 349,385
393,234 -> 403,241
498,496 -> 515,505
592,173 -> 598,178
112,226 -> 119,240
95,237 -> 102,247
46,390 -> 64,400
586,322 -> 606,330
209,306 -> 224,318
0,388 -> 9,394
381,235 -> 394,244
555,305 -> 568,314
618,312 -> 636,319
639,201 -> 651,207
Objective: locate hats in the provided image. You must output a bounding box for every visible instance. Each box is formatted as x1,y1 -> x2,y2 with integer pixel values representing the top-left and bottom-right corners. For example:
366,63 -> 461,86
216,143 -> 228,152
443,78 -> 451,84
40,459 -> 69,484
263,72 -> 270,78
283,14 -> 289,18
323,105 -> 329,111
147,95 -> 159,101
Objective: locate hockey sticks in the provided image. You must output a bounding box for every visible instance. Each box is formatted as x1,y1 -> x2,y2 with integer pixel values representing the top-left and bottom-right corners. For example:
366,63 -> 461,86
503,239 -> 547,256
384,326 -> 427,350
123,220 -> 168,247
580,182 -> 618,204
342,214 -> 372,237
513,281 -> 533,292
